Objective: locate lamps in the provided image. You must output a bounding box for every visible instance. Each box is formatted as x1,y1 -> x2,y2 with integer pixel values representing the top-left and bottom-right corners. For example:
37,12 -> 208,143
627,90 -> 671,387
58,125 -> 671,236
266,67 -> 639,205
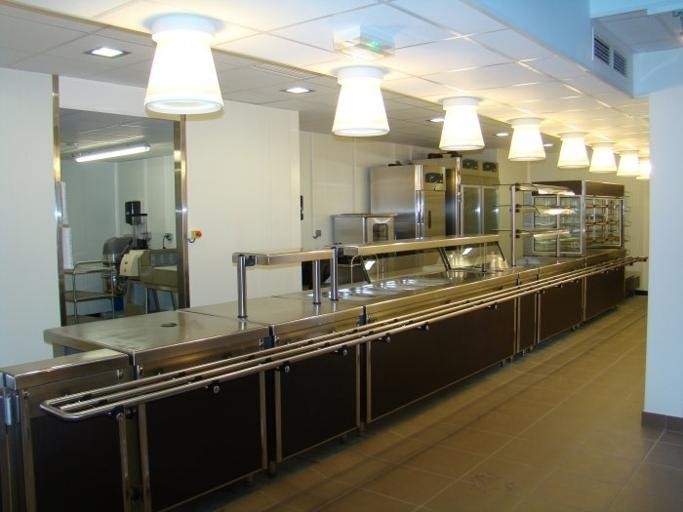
616,149 -> 650,180
556,131 -> 591,170
330,65 -> 390,139
71,144 -> 151,163
438,97 -> 486,152
507,117 -> 547,162
589,142 -> 618,175
142,14 -> 226,123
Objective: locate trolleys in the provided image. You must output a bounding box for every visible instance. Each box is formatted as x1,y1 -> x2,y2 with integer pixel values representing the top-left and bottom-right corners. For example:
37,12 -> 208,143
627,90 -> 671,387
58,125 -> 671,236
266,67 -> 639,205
64,257 -> 119,324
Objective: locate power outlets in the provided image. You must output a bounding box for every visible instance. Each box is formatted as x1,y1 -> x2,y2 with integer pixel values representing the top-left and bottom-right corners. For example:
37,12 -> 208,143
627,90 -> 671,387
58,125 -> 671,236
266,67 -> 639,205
165,233 -> 170,239
